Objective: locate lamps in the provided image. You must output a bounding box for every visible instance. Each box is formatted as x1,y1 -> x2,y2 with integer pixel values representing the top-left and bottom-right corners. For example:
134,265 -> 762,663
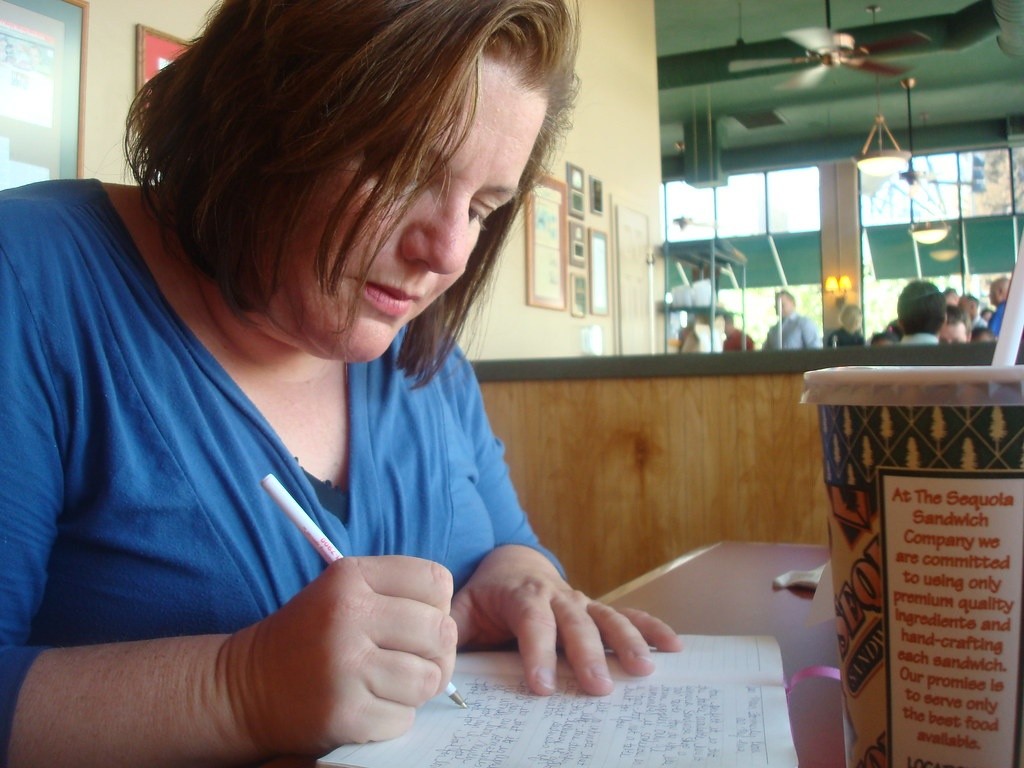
851,5 -> 949,245
826,276 -> 852,310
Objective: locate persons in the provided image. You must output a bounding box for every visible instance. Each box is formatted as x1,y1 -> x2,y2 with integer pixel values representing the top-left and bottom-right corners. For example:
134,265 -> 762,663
827,305 -> 865,346
762,291 -> 823,349
872,276 -> 1011,345
723,315 -> 755,353
0,0 -> 683,768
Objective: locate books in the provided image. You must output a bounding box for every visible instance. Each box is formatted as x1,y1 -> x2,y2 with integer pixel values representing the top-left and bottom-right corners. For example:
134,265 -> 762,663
317,634 -> 799,768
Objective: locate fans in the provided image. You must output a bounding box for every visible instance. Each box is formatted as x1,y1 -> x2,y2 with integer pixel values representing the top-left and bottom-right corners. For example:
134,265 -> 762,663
730,0 -> 933,90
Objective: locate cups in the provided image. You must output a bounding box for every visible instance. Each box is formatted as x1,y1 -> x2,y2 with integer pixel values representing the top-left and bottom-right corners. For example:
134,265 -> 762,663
800,366 -> 1023,768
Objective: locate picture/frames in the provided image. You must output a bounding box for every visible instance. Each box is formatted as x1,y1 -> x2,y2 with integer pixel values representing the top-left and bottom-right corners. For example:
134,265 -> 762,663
134,23 -> 193,94
524,163 -> 609,317
0,1 -> 89,190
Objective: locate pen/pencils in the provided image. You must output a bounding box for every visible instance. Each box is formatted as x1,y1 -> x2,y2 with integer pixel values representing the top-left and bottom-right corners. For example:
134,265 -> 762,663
260,473 -> 468,710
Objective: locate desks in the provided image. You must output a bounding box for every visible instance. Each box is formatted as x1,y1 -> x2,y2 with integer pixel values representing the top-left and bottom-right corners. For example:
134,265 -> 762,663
252,541 -> 847,768
